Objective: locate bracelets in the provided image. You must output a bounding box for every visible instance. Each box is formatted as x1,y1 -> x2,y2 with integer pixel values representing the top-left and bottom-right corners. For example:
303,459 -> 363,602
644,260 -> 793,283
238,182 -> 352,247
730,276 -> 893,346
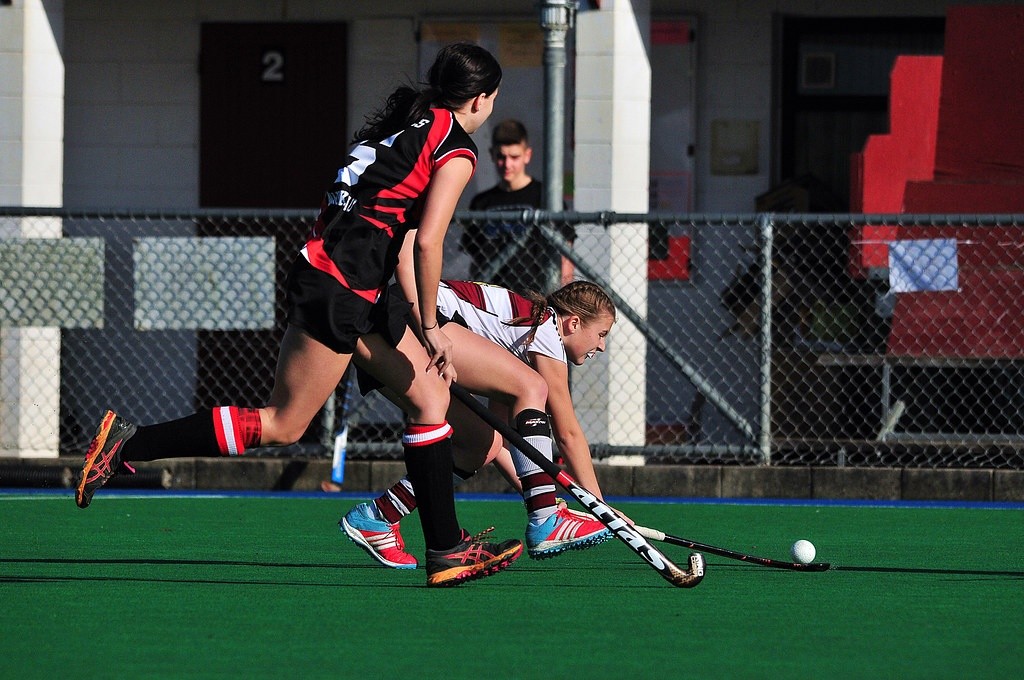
421,319 -> 437,330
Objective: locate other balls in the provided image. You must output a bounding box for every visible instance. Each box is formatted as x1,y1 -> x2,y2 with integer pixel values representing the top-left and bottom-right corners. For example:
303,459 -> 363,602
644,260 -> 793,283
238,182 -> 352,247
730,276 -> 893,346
787,537 -> 820,567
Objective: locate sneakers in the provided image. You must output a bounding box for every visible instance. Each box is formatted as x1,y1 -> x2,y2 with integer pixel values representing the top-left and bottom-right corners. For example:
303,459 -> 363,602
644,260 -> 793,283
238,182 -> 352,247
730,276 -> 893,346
74,410 -> 136,508
525,501 -> 615,560
338,503 -> 419,570
425,527 -> 524,588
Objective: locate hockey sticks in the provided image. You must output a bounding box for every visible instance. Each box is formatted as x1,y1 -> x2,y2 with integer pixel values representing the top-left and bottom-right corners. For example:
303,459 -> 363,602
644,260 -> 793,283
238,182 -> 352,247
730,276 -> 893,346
562,505 -> 833,576
446,378 -> 710,591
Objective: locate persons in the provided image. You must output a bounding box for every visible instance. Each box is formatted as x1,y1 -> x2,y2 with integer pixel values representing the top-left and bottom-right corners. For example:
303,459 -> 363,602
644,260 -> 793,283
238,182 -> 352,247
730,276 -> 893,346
75,43 -> 621,588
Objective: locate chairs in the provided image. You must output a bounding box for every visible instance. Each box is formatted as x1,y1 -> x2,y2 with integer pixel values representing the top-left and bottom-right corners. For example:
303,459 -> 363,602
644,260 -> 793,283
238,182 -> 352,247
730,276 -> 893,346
814,0 -> 1024,447
